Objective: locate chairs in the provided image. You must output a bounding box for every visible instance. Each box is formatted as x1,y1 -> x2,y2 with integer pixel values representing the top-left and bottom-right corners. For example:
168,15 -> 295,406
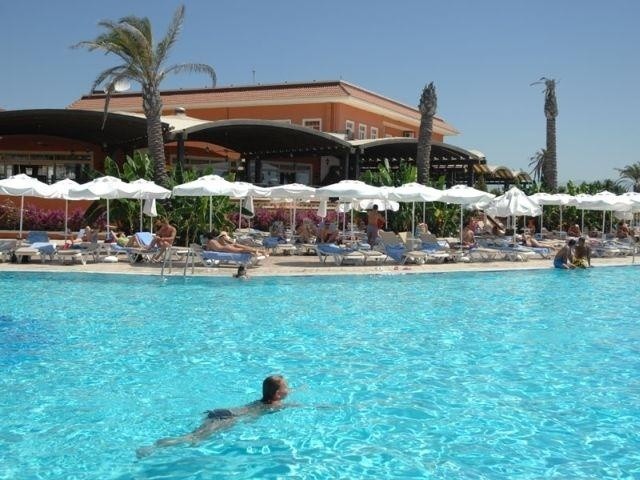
1,217 -> 640,271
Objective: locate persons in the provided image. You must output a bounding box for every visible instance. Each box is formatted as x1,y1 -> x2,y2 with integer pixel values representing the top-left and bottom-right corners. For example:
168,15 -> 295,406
204,204 -> 387,280
68,217 -> 176,257
135,373 -> 331,460
461,217 -> 640,270
